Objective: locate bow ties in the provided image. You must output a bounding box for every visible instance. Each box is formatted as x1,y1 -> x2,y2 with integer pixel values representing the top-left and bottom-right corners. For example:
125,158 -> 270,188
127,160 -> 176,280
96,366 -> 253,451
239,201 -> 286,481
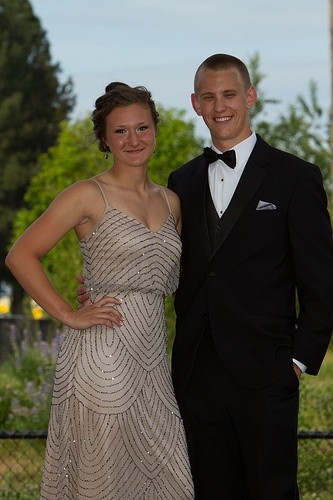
203,147 -> 237,170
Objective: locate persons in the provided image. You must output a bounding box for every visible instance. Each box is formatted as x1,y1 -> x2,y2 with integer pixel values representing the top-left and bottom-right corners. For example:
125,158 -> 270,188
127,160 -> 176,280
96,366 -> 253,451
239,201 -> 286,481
77,54 -> 333,500
6,83 -> 194,500
